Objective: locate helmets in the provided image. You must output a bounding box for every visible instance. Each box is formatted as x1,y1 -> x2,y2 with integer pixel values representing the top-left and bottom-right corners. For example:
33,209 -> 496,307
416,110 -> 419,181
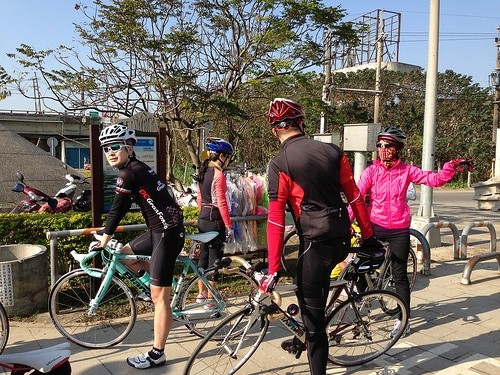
376,126 -> 407,153
98,124 -> 137,146
206,137 -> 233,155
267,97 -> 304,130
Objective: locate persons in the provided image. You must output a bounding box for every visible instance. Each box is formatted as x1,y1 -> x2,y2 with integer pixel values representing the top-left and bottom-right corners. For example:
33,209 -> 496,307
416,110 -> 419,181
348,124 -> 470,338
262,95 -> 372,374
88,124 -> 185,370
195,138 -> 235,309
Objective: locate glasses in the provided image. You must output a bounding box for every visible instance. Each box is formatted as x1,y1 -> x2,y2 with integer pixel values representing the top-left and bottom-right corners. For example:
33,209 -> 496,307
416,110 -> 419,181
376,143 -> 397,149
103,144 -> 131,153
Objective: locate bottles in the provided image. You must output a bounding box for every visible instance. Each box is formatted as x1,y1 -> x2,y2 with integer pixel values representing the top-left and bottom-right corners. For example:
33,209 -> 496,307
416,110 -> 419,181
287,303 -> 304,326
138,269 -> 151,288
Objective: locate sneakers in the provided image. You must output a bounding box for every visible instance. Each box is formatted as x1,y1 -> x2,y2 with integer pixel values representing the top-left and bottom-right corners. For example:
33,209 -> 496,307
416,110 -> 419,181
389,319 -> 410,339
196,291 -> 208,304
203,300 -> 221,310
350,297 -> 378,310
127,351 -> 166,369
138,291 -> 152,302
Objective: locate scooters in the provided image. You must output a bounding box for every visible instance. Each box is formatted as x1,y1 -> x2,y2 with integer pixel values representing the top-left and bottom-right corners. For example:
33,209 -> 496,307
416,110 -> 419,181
9,164 -> 91,215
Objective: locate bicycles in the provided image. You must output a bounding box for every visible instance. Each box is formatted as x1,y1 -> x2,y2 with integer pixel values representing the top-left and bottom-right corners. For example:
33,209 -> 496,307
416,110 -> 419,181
48,231 -> 268,349
0,302 -> 11,355
184,222 -> 418,375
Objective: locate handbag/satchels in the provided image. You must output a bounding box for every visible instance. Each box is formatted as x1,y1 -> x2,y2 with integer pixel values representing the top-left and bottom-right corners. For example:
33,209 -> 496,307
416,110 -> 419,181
353,252 -> 386,273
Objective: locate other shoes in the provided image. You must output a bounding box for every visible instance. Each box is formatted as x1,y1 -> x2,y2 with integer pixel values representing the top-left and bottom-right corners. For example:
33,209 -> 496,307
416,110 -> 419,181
281,339 -> 303,351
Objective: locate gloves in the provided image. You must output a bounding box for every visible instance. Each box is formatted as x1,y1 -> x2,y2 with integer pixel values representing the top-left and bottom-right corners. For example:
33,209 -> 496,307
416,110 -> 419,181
225,226 -> 234,243
350,226 -> 355,238
362,236 -> 384,250
451,158 -> 474,173
